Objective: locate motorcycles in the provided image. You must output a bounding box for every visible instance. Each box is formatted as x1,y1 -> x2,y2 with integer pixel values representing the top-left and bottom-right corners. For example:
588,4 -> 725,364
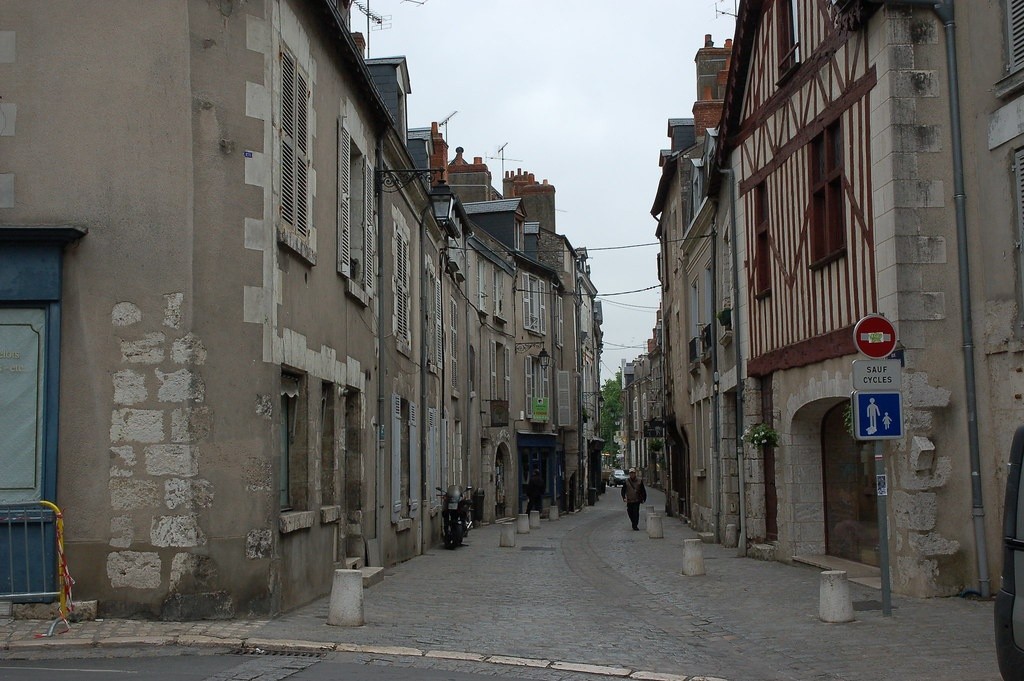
435,484 -> 475,550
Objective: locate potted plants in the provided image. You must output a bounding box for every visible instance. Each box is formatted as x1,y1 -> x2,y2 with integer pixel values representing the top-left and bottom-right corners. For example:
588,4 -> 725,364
650,439 -> 664,451
718,307 -> 731,325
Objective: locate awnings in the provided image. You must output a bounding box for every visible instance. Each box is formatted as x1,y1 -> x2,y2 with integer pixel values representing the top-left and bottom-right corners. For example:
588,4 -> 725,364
516,430 -> 558,436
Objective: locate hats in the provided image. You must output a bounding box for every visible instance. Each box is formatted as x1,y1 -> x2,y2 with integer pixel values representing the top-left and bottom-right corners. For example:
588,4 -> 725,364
629,468 -> 636,472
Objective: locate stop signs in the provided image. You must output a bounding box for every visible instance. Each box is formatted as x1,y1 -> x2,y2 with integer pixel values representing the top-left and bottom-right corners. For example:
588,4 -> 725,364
854,313 -> 899,360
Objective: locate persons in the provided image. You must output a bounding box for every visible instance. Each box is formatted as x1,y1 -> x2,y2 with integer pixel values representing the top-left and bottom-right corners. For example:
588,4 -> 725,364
525,470 -> 546,520
621,468 -> 646,531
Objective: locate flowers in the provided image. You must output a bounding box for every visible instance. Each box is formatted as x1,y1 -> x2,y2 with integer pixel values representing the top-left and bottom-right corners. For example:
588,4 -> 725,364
741,422 -> 780,450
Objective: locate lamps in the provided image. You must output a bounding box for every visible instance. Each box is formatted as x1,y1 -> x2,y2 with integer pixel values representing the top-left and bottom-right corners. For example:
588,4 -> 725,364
515,340 -> 550,370
374,162 -> 456,225
584,388 -> 604,407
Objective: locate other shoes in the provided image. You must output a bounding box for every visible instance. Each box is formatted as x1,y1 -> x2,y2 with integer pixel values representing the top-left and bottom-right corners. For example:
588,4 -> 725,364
632,524 -> 639,530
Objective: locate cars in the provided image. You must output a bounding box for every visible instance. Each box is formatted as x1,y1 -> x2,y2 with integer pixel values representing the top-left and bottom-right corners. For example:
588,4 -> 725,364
608,469 -> 629,488
